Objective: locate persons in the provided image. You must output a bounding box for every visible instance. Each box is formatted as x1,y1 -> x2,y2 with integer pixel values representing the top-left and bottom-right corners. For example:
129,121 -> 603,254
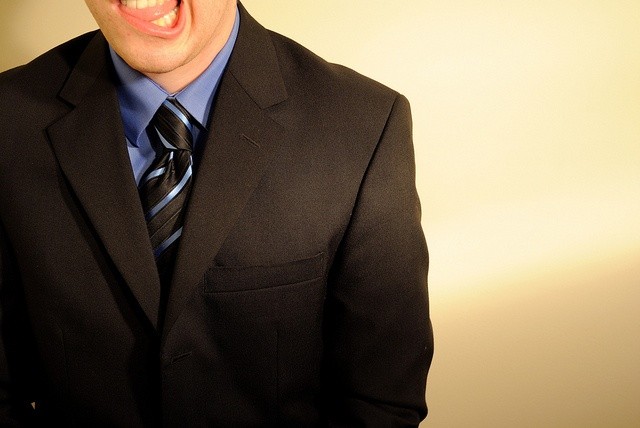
0,0 -> 434,428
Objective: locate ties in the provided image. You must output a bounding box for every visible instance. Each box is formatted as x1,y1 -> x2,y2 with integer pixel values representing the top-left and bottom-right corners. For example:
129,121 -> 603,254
136,94 -> 195,268
